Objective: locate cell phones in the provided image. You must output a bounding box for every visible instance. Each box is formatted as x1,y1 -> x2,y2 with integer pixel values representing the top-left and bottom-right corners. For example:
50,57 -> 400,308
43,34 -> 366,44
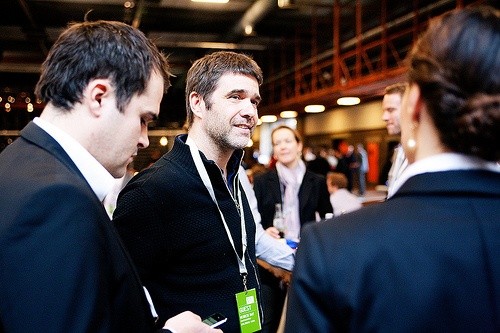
204,312 -> 227,328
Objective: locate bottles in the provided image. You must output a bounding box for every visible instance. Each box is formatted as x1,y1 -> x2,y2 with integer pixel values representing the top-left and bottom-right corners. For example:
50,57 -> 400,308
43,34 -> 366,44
323,213 -> 334,223
273,203 -> 286,238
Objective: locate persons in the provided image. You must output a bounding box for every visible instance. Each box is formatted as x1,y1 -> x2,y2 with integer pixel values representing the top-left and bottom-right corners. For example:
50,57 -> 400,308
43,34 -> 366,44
301,140 -> 382,197
285,4 -> 500,332
2,17 -> 173,331
251,124 -> 336,332
382,82 -> 412,199
111,49 -> 272,332
326,171 -> 362,216
